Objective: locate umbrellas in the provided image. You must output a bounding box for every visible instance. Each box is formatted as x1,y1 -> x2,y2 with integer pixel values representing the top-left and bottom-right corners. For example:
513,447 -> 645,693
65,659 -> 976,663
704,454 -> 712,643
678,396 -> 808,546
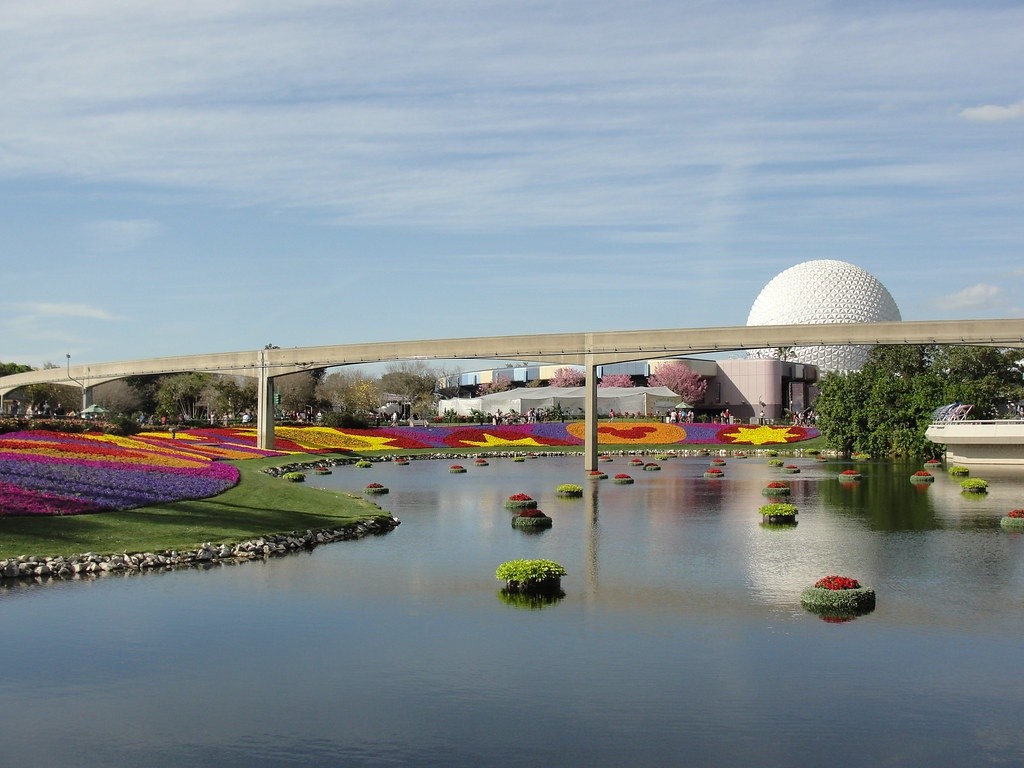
675,402 -> 694,408
81,404 -> 110,412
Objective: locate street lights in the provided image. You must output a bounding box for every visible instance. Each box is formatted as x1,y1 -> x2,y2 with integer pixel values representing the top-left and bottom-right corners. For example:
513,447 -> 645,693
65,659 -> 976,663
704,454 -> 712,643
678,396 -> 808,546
306,404 -> 312,422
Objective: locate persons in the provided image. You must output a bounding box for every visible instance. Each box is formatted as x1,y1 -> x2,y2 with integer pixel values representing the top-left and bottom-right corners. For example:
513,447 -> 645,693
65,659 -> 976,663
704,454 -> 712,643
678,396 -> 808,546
281,410 -> 322,423
381,411 -> 421,427
721,409 -> 730,424
496,409 -> 518,424
525,408 -> 549,422
793,411 -> 815,426
666,409 -> 692,423
243,412 -> 254,427
139,413 -> 185,427
11,400 -> 104,419
211,412 -> 228,426
760,411 -> 765,425
609,409 -> 615,423
